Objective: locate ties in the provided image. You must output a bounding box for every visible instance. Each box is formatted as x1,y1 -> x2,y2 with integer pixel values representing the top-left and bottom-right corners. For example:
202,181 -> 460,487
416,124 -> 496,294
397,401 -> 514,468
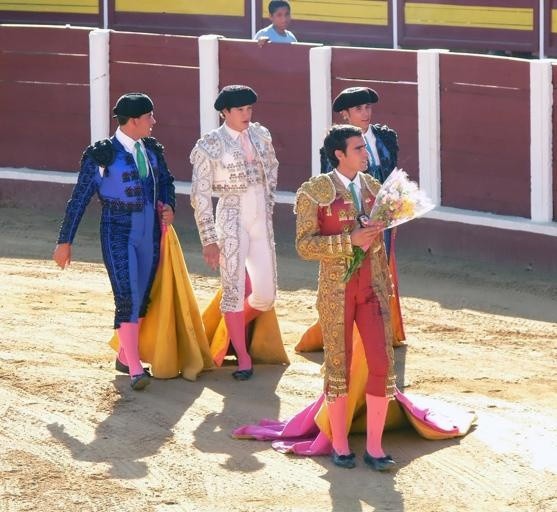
239,132 -> 254,164
349,183 -> 360,215
134,142 -> 148,179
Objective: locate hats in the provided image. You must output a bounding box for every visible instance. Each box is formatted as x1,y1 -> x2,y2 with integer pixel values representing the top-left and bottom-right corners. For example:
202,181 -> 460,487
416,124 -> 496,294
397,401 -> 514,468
114,92 -> 155,118
330,87 -> 380,112
214,85 -> 257,110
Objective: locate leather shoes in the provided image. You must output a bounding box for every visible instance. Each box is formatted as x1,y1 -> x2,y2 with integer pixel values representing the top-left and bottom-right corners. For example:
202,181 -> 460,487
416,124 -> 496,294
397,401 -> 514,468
331,448 -> 356,469
115,356 -> 152,378
362,448 -> 397,472
129,367 -> 151,391
231,354 -> 254,380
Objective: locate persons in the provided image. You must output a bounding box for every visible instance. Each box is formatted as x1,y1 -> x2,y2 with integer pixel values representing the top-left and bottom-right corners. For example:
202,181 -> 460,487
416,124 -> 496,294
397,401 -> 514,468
50,91 -> 175,391
291,125 -> 397,475
318,85 -> 400,267
254,1 -> 298,48
187,84 -> 279,381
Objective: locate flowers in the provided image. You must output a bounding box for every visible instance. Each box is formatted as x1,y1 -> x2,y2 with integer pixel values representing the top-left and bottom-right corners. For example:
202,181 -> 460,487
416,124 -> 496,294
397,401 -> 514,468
343,167 -> 434,282
